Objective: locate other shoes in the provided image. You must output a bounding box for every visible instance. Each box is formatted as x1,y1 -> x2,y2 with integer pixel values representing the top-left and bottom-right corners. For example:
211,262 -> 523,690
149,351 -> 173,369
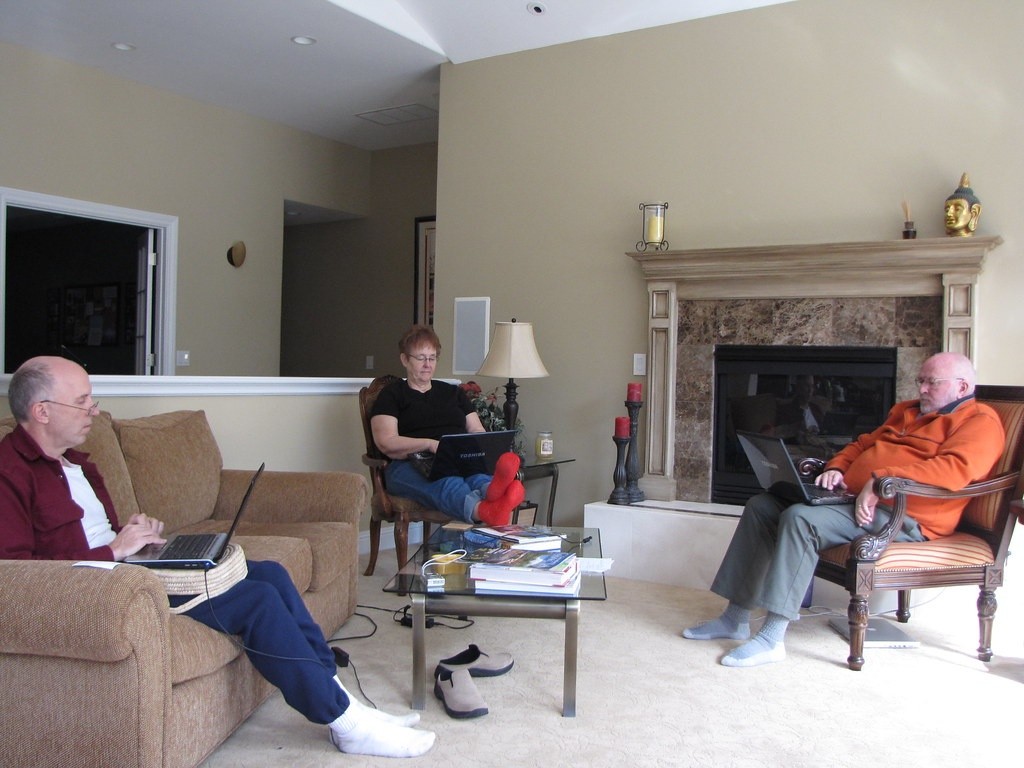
434,644 -> 515,678
434,668 -> 488,719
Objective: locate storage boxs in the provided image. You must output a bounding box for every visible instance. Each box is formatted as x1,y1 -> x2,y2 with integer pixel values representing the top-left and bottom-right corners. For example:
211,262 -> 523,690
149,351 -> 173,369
507,500 -> 538,527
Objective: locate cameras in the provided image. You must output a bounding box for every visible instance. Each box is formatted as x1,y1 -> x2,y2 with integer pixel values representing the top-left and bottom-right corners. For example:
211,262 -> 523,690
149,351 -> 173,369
420,572 -> 445,587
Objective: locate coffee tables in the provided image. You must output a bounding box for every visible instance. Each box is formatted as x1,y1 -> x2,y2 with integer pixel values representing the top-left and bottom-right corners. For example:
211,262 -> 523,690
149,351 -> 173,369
383,519 -> 608,718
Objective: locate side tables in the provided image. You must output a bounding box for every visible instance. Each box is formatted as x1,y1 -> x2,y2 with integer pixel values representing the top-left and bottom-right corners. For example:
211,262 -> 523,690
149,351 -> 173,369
512,454 -> 576,527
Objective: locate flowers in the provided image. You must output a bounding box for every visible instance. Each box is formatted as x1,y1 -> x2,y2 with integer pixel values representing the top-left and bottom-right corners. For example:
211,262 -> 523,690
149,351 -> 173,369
459,381 -> 529,456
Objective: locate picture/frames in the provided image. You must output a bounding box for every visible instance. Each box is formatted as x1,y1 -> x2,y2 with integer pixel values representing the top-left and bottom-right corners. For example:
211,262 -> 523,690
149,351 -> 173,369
414,215 -> 436,326
46,280 -> 121,347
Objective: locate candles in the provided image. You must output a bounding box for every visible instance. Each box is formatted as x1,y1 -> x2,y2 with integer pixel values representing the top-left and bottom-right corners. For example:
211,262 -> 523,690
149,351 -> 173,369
627,383 -> 642,402
615,416 -> 630,439
648,217 -> 663,243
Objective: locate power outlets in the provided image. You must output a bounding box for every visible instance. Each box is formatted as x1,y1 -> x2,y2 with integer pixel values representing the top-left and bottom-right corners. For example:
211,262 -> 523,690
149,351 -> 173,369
366,356 -> 374,369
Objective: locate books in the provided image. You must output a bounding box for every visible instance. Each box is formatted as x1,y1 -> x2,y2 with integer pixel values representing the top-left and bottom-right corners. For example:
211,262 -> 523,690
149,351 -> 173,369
464,524 -> 567,551
460,547 -> 581,595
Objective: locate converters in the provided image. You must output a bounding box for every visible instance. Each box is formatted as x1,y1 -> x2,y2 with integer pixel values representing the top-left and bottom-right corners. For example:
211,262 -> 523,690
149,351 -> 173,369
330,646 -> 349,668
401,615 -> 434,628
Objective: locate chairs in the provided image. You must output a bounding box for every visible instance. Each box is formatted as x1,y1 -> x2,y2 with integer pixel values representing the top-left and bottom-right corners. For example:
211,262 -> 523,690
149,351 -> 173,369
801,385 -> 1024,671
359,375 -> 455,597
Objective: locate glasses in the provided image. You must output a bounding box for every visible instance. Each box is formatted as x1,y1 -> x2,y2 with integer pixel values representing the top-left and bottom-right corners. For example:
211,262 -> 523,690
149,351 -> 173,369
403,352 -> 440,364
41,400 -> 101,417
915,378 -> 965,389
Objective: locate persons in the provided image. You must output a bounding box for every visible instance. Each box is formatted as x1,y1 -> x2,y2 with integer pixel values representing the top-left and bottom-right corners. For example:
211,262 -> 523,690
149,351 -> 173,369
774,375 -> 826,436
944,172 -> 981,238
0,356 -> 435,758
684,352 -> 1006,666
370,324 -> 526,526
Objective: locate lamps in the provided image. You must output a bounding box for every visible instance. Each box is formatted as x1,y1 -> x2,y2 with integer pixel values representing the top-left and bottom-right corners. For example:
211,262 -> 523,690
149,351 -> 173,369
474,318 -> 551,430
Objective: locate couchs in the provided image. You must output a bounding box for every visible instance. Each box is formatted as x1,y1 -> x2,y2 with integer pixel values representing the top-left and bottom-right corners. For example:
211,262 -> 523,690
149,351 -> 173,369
0,409 -> 372,768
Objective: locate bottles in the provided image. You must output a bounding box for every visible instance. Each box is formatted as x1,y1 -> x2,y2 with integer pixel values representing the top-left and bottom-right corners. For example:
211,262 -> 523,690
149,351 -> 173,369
535,430 -> 554,460
902,221 -> 917,239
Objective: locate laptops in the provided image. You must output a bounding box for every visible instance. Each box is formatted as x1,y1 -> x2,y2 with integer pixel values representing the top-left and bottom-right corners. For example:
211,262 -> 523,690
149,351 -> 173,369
734,428 -> 857,506
406,430 -> 517,483
828,618 -> 921,648
122,462 -> 265,570
